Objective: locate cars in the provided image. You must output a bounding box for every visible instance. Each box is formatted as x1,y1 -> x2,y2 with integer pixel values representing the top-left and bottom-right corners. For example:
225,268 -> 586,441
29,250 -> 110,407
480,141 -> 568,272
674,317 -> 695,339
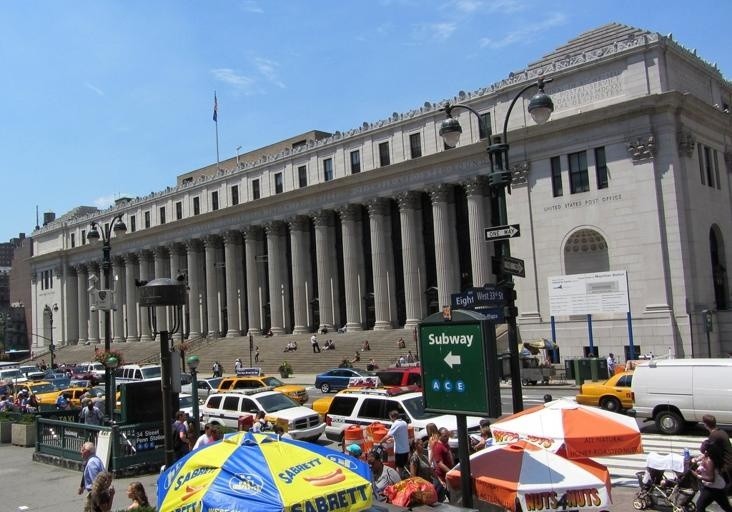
182,376 -> 219,392
177,394 -> 204,416
377,368 -> 424,397
0,359 -> 121,411
314,368 -> 377,393
312,377 -> 425,415
575,372 -> 634,411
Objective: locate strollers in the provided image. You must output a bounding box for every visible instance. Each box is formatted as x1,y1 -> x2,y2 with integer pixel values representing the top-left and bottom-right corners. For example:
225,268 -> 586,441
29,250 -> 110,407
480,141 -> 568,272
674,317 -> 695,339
634,449 -> 703,512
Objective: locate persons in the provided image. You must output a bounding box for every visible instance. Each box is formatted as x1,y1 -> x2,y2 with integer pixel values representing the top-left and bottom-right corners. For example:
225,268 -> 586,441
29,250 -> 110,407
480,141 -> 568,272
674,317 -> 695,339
235,358 -> 242,374
607,352 -> 616,377
702,414 -> 732,482
345,410 -> 491,505
251,410 -> 292,444
338,325 -> 347,334
323,339 -> 335,351
33,357 -> 74,380
311,334 -> 321,353
396,337 -> 420,368
79,440 -> 155,511
254,346 -> 260,363
321,325 -> 328,335
55,370 -> 105,426
339,339 -> 380,372
689,439 -> 732,512
167,409 -> 224,456
285,341 -> 297,353
0,380 -> 38,412
212,358 -> 224,378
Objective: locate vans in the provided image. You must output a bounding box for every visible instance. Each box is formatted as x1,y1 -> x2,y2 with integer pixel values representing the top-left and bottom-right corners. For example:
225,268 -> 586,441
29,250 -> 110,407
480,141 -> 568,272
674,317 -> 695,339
630,358 -> 732,436
113,365 -> 162,382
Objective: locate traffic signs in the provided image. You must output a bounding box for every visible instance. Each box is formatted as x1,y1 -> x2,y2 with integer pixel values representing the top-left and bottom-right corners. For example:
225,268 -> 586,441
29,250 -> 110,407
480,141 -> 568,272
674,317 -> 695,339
494,254 -> 526,277
421,322 -> 489,417
484,222 -> 519,241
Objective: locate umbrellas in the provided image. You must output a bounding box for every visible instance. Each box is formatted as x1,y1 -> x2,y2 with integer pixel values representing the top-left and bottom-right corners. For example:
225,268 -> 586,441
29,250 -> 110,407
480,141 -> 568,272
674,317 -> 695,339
450,397 -> 641,510
157,426 -> 373,512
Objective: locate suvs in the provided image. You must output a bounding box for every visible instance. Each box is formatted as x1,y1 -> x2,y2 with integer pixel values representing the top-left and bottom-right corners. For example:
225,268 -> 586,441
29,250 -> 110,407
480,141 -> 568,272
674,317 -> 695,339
203,392 -> 326,444
217,376 -> 307,404
326,392 -> 489,441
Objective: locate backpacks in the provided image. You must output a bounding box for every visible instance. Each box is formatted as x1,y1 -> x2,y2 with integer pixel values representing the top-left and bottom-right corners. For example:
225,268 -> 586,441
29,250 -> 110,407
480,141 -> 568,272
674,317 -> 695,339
173,423 -> 183,450
259,420 -> 271,432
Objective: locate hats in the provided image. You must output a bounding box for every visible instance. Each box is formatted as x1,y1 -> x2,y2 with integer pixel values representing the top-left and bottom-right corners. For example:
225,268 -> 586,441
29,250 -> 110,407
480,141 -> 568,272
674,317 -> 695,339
346,444 -> 362,456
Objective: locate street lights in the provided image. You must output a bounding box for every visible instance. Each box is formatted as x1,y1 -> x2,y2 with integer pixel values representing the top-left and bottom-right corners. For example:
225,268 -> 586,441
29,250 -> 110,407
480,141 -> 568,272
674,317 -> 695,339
43,303 -> 57,367
437,78 -> 565,410
84,217 -> 136,355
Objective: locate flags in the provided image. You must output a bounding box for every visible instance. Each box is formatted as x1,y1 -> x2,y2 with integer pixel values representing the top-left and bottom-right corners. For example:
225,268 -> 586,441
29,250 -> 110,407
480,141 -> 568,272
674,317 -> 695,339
213,95 -> 218,121
